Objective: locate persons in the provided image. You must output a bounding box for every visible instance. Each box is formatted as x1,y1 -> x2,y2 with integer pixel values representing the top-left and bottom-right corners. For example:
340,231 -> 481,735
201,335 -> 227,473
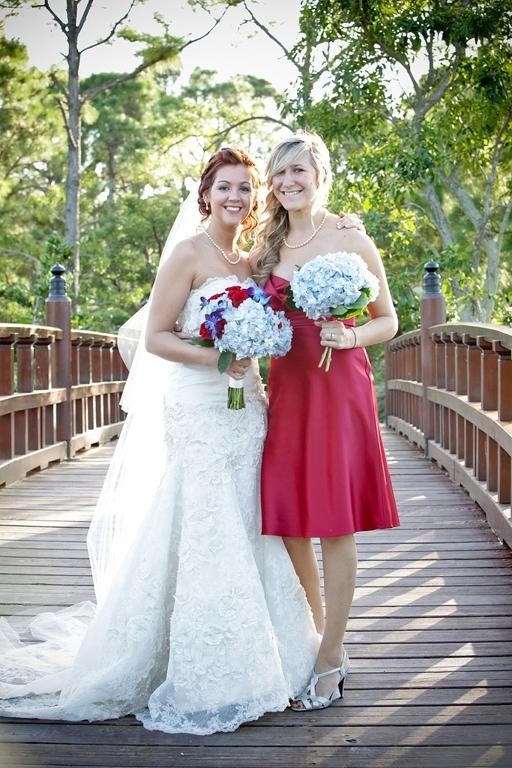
247,129 -> 399,713
1,145 -> 367,735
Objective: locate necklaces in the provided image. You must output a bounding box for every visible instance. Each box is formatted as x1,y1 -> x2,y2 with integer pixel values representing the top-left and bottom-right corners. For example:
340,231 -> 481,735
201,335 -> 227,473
283,210 -> 329,249
204,229 -> 241,265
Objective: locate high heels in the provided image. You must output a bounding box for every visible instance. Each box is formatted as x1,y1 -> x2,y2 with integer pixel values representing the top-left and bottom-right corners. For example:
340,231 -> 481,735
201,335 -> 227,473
291,645 -> 350,711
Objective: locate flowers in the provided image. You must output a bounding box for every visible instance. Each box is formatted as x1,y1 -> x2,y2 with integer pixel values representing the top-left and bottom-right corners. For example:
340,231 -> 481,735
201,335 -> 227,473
200,286 -> 295,410
285,251 -> 381,374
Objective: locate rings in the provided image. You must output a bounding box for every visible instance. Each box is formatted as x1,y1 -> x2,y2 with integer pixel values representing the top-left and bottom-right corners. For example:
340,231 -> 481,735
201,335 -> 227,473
358,219 -> 363,224
331,333 -> 334,341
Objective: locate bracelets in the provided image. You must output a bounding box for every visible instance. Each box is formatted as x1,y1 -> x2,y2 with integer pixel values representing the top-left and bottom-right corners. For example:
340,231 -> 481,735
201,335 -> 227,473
351,327 -> 357,350
